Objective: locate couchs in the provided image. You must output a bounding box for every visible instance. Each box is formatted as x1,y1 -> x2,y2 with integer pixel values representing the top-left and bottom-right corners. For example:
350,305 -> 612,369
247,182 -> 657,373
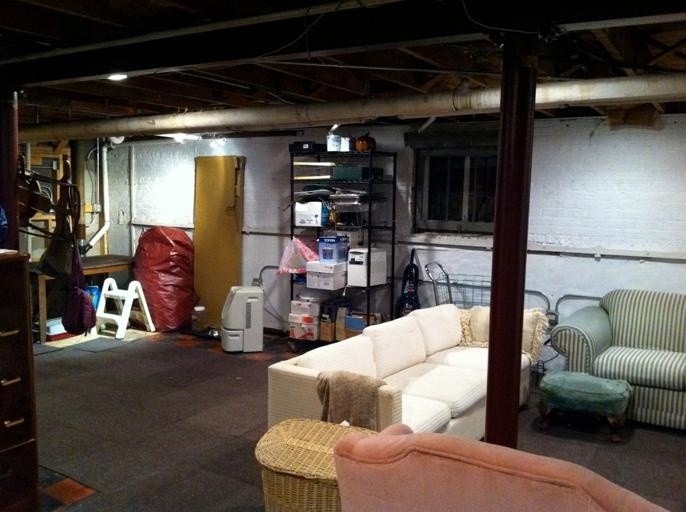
265,300 -> 548,440
548,289 -> 683,432
333,425 -> 670,512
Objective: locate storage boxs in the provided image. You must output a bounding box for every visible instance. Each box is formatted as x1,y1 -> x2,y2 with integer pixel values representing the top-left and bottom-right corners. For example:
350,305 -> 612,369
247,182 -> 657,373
254,415 -> 379,512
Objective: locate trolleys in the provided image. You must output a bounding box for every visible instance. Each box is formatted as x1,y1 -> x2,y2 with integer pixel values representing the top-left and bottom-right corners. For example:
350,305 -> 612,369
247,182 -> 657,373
425,262 -> 492,305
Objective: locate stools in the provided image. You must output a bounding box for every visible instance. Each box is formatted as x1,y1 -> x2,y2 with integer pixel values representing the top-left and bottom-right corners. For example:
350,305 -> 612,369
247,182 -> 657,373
538,368 -> 629,444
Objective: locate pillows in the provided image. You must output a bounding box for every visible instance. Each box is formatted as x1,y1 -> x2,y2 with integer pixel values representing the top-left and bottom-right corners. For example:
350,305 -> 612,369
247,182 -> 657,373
460,306 -> 548,361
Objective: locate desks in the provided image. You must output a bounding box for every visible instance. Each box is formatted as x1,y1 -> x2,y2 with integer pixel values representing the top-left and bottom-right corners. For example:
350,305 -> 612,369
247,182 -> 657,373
30,255 -> 137,342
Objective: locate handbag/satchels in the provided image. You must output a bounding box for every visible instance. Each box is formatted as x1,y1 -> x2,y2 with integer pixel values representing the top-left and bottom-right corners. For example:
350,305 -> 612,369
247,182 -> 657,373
37,178 -> 74,279
62,242 -> 97,334
396,294 -> 419,318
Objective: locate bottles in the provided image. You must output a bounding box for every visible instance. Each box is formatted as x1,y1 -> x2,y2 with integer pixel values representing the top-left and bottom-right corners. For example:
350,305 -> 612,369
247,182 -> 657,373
192,306 -> 206,336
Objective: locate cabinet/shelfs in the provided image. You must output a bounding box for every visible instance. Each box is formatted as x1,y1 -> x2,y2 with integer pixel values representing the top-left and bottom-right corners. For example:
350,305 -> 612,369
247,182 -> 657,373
2,250 -> 40,511
290,138 -> 397,355
